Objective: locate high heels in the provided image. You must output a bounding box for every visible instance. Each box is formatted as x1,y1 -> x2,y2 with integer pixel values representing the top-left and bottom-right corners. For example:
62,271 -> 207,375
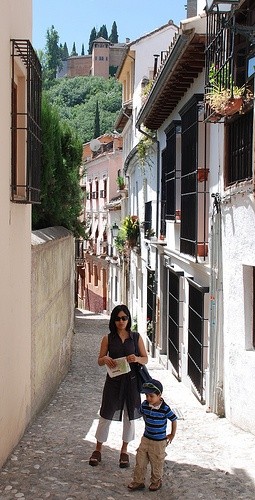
120,453 -> 130,467
89,451 -> 101,465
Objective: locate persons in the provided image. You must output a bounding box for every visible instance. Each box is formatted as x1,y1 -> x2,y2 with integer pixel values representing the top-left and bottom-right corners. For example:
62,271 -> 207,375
127,378 -> 178,491
89,305 -> 149,467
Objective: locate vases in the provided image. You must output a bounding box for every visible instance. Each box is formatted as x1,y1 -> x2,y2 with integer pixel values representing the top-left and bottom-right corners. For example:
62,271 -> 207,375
196,242 -> 208,256
197,167 -> 209,182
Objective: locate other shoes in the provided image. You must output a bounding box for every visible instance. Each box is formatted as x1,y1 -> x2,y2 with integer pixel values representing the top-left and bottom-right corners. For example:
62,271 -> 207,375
149,481 -> 162,490
128,482 -> 145,489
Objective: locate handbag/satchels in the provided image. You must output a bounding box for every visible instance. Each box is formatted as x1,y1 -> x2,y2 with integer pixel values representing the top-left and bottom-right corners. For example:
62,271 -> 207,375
134,363 -> 152,393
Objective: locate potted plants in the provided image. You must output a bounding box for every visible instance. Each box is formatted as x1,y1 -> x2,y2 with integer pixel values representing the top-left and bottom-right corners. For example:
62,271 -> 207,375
115,215 -> 140,252
206,63 -> 254,115
116,177 -> 125,190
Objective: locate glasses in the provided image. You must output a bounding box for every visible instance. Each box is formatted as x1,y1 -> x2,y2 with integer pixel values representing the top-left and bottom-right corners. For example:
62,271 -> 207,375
114,316 -> 128,321
143,383 -> 160,391
